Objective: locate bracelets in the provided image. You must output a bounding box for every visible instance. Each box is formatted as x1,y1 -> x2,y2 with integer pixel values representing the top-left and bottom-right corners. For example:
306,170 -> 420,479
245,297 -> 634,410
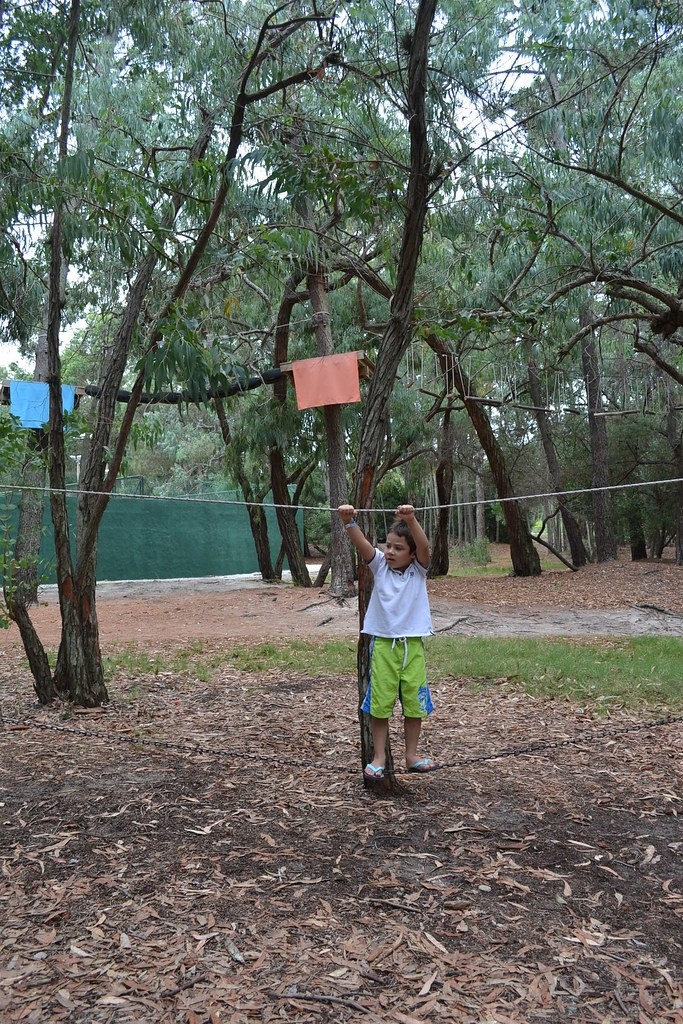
344,521 -> 358,529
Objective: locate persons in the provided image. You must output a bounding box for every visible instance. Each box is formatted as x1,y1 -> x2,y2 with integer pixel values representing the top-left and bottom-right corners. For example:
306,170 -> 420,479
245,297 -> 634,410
337,504 -> 438,780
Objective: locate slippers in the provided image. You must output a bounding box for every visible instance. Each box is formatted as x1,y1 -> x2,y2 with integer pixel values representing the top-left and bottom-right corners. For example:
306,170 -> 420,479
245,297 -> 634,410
407,758 -> 437,772
363,762 -> 387,781
